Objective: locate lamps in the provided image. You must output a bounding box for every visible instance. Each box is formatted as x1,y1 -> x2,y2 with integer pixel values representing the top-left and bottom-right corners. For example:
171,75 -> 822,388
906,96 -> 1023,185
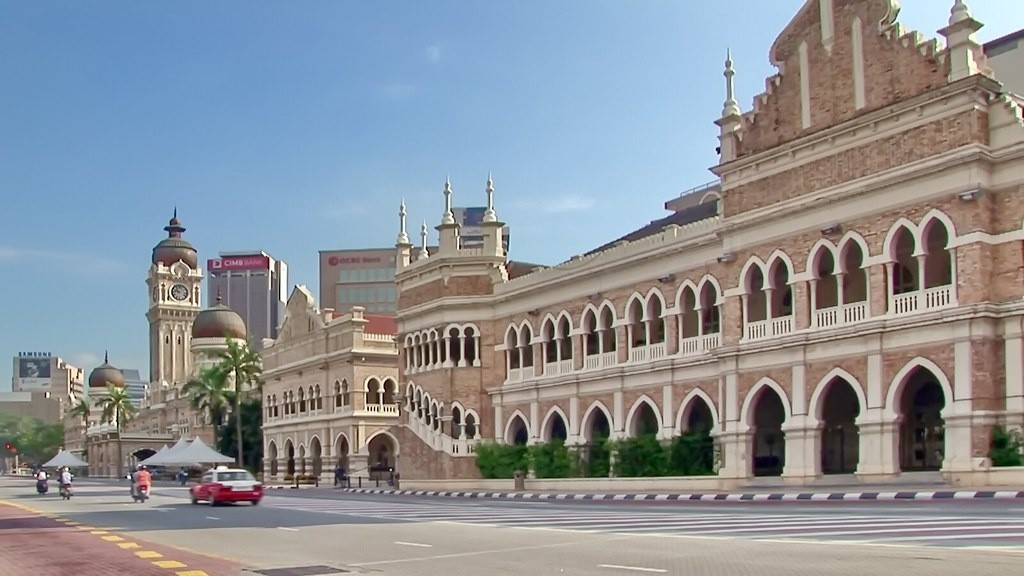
959,189 -> 982,201
820,224 -> 841,235
587,292 -> 602,302
717,253 -> 737,264
527,308 -> 539,315
657,273 -> 673,283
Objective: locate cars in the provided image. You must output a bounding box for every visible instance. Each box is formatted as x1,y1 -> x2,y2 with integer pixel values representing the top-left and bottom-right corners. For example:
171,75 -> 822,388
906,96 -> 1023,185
190,465 -> 264,507
124,468 -> 201,480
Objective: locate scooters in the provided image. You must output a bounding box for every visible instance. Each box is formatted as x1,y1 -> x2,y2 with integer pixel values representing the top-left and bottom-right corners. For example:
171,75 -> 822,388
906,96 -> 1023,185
34,473 -> 50,495
59,474 -> 75,500
132,477 -> 149,503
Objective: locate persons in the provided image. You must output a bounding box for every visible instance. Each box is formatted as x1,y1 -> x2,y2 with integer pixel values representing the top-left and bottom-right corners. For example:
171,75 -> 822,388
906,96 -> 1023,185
36,466 -> 71,496
131,464 -> 152,500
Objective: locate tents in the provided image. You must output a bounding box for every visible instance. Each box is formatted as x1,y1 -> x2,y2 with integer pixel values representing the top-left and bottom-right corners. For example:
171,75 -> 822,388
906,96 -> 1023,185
136,435 -> 235,473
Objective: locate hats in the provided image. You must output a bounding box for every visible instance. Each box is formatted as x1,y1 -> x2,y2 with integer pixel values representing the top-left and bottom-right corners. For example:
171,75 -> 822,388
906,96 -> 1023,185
141,465 -> 147,469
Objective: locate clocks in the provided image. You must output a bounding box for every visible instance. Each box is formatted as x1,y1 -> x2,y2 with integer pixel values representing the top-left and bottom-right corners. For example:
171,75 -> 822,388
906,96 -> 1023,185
171,284 -> 188,301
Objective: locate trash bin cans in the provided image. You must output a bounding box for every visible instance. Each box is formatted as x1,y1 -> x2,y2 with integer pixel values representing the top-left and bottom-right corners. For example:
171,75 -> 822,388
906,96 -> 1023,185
512,470 -> 525,491
